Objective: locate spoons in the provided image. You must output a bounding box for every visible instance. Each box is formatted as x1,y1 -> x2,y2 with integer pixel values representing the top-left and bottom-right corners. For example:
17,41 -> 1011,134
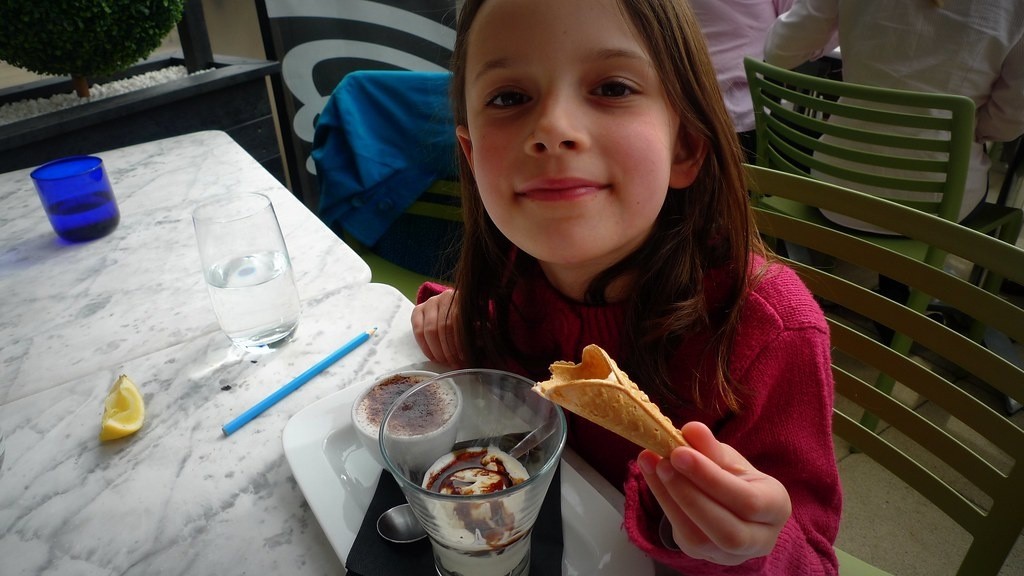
378,418 -> 561,543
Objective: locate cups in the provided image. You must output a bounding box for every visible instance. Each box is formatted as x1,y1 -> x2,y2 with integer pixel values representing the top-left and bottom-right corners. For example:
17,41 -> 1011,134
378,368 -> 566,576
192,192 -> 301,346
30,155 -> 121,243
351,369 -> 463,473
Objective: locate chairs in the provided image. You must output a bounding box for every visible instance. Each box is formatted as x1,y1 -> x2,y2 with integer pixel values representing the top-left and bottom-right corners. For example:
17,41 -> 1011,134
738,159 -> 1022,575
741,48 -> 1024,449
327,69 -> 488,305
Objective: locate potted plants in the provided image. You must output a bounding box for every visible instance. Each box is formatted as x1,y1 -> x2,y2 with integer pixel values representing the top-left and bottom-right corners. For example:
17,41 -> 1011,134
0,0 -> 300,198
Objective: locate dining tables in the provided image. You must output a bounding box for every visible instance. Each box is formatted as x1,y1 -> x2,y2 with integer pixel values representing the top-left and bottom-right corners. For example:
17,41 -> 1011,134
1,126 -> 666,575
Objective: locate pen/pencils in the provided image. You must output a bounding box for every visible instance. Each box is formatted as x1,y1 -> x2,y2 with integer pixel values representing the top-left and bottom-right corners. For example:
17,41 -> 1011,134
220,325 -> 379,439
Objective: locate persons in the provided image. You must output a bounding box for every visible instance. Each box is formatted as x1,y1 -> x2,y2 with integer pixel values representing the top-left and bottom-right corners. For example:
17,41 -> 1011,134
762,0 -> 1024,358
403,0 -> 845,576
682,0 -> 845,163
